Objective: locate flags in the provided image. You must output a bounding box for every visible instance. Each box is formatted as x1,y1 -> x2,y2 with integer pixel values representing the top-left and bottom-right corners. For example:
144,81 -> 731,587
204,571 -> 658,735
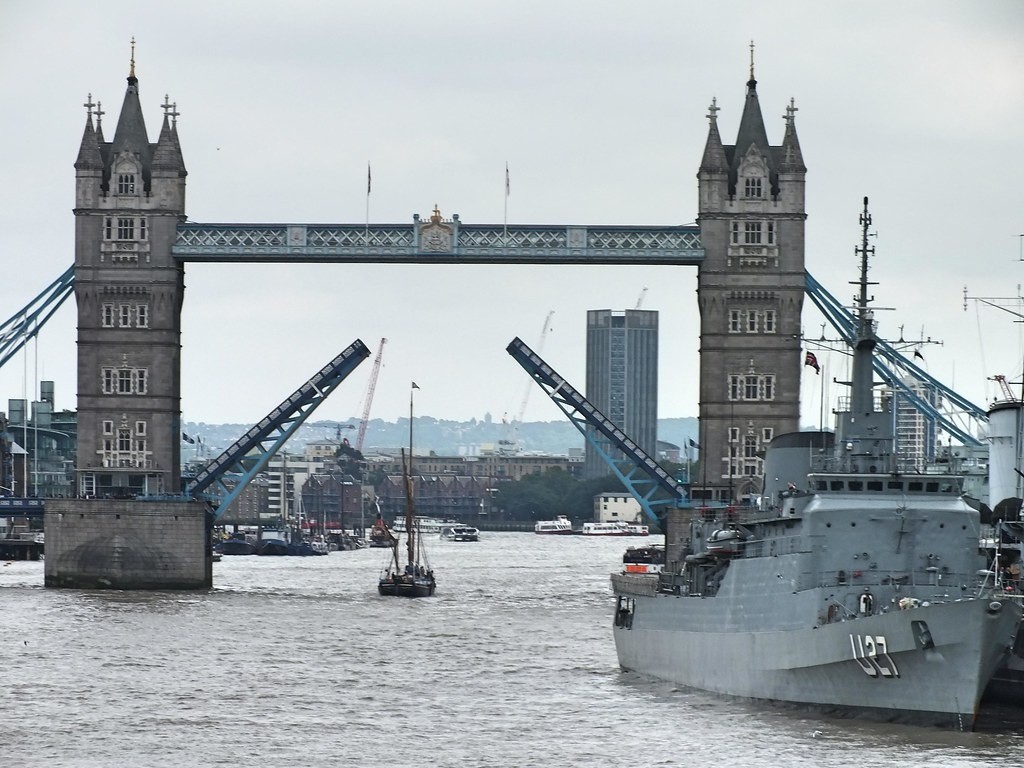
805,350 -> 820,374
690,438 -> 703,449
183,433 -> 195,444
914,349 -> 923,360
684,440 -> 688,458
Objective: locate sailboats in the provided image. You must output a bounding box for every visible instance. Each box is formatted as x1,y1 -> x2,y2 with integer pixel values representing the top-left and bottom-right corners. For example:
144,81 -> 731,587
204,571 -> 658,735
378,397 -> 438,603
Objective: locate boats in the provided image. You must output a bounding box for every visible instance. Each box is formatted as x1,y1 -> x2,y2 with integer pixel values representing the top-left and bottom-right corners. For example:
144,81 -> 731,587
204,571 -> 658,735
393,508 -> 484,554
607,195 -> 1024,734
534,514 -> 649,537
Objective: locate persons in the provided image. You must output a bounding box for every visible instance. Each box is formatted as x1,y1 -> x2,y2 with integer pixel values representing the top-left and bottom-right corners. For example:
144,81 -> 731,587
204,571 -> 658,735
788,482 -> 797,494
992,564 -> 1013,587
385,565 -> 435,584
922,458 -> 928,471
